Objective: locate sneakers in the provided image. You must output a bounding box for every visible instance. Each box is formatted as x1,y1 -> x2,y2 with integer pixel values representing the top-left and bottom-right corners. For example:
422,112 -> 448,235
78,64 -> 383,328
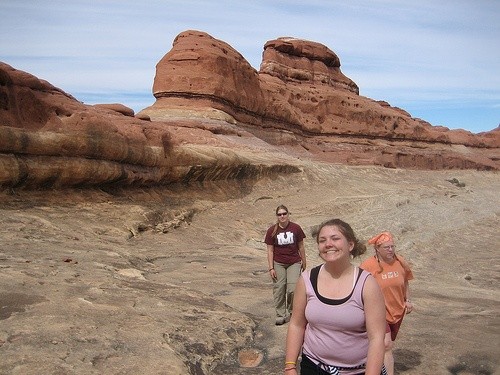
276,318 -> 286,324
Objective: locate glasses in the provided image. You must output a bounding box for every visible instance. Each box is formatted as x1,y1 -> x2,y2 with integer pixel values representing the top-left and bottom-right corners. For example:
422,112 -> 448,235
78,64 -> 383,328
277,211 -> 287,216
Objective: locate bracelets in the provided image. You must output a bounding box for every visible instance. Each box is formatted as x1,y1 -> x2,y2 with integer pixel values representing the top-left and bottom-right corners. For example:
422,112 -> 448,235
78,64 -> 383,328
269,268 -> 274,271
284,362 -> 296,371
405,298 -> 412,302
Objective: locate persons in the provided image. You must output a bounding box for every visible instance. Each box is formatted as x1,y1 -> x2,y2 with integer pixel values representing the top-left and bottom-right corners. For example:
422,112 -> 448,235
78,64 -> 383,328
265,205 -> 306,325
359,232 -> 412,375
284,219 -> 386,375
